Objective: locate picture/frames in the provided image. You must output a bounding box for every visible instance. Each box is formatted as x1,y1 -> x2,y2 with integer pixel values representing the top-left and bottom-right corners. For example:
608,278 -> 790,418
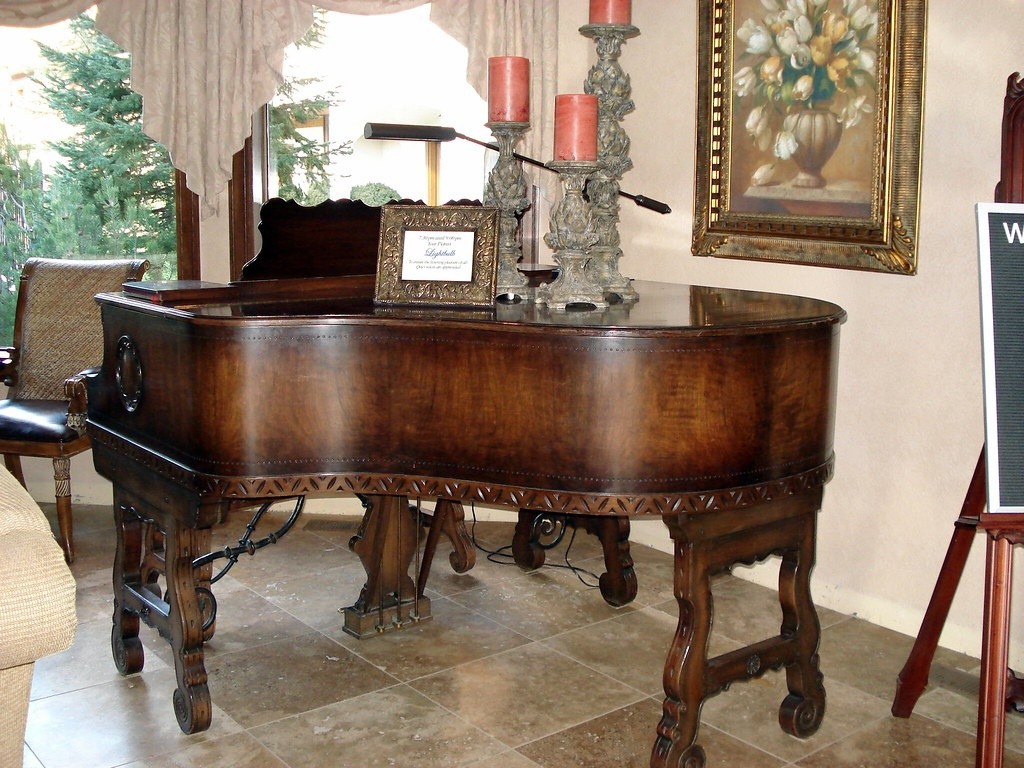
374,203 -> 502,307
693,0 -> 924,275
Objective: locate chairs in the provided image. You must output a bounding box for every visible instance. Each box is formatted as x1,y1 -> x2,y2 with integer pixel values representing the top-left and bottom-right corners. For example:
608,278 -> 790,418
0,256 -> 152,563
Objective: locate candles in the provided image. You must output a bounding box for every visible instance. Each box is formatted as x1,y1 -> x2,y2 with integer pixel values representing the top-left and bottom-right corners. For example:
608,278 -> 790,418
589,0 -> 631,24
554,92 -> 599,162
487,55 -> 529,122
